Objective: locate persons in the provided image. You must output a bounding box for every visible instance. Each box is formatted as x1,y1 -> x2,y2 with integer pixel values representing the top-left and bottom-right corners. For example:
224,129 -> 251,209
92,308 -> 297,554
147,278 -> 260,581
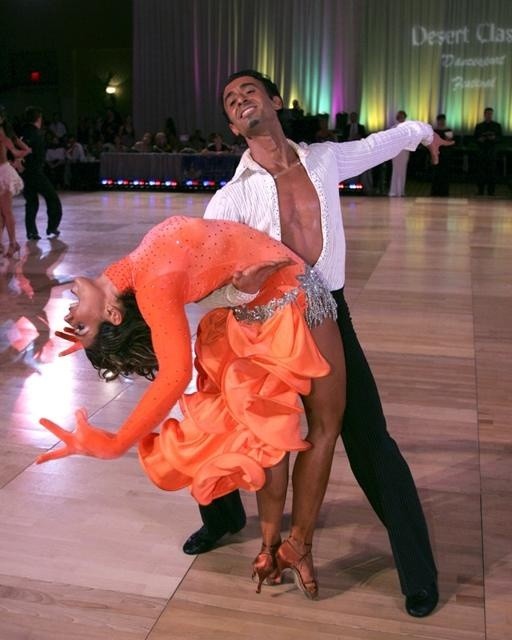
6,105 -> 63,237
101,109 -> 234,153
280,98 -> 510,196
12,114 -> 25,140
0,105 -> 33,260
20,234 -> 77,359
44,112 -> 105,190
180,67 -> 455,625
32,213 -> 348,600
0,254 -> 35,382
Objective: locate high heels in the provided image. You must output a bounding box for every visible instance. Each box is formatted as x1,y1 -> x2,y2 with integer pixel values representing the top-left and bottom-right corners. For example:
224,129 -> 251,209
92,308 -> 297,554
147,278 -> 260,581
252,536 -> 319,600
4,243 -> 20,258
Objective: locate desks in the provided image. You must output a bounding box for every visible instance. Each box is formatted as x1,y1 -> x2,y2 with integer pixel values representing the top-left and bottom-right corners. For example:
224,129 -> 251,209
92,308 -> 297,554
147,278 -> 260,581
95,151 -> 241,194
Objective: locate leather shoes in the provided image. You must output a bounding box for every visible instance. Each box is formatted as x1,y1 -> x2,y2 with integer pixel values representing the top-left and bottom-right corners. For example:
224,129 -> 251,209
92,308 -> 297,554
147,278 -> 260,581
27,233 -> 41,240
405,577 -> 439,617
183,515 -> 246,555
47,230 -> 60,235
